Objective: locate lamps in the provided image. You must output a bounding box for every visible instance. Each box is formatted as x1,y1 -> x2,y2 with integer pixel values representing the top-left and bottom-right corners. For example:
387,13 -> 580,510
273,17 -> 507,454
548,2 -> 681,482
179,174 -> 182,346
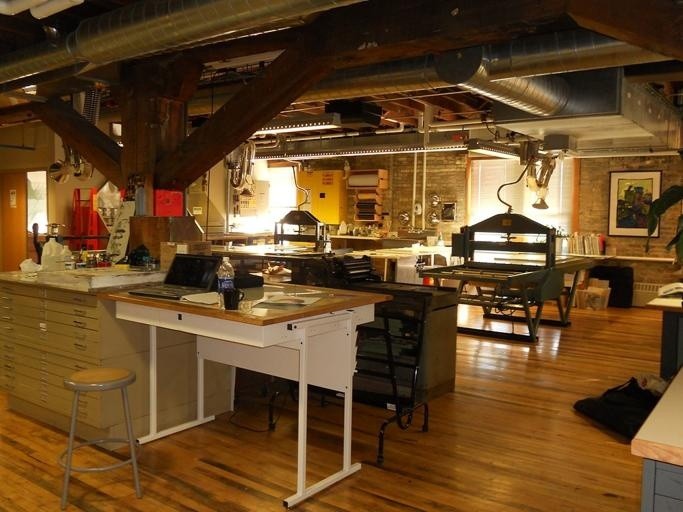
255,132 -> 520,160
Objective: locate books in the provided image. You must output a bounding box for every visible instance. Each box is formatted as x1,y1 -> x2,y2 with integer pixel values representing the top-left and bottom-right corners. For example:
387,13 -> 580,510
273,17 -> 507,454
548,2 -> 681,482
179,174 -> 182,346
179,292 -> 220,305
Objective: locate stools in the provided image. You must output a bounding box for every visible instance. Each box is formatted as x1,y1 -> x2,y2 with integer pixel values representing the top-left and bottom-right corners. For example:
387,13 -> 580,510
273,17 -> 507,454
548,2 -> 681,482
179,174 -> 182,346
59,369 -> 142,509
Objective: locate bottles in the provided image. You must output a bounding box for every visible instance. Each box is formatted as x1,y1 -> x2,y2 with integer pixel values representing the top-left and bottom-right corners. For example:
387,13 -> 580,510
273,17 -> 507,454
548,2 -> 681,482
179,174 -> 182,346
340,221 -> 354,235
80,244 -> 88,262
61,245 -> 72,269
218,256 -> 235,307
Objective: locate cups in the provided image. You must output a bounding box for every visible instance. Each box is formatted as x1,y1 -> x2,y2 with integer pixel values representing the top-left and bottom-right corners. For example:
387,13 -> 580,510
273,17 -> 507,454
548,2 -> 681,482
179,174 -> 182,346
223,288 -> 244,310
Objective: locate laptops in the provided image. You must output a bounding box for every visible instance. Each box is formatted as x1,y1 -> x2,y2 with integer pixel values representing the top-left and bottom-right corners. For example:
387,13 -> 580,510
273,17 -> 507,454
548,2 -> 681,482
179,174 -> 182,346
128,253 -> 223,300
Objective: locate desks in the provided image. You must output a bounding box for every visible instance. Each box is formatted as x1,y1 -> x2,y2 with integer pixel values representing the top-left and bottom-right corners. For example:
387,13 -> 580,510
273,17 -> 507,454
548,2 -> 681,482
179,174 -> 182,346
419,253 -> 593,344
177,236 -> 463,282
104,283 -> 388,510
632,289 -> 683,512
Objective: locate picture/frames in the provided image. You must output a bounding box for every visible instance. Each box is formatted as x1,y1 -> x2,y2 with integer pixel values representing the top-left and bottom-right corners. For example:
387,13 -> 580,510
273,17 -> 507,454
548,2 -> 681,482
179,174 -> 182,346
608,170 -> 662,238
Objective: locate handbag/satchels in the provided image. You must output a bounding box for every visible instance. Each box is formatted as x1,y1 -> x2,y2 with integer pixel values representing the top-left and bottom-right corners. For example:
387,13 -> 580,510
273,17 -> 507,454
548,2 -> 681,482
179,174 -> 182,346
573,378 -> 660,439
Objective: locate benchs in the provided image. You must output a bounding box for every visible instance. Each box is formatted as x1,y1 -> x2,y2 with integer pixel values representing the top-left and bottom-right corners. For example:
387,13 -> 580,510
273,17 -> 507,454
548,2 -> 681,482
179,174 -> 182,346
264,287 -> 431,464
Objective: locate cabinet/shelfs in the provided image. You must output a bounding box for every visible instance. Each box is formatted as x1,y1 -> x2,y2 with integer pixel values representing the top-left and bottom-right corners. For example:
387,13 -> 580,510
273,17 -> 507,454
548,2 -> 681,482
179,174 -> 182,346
335,282 -> 459,400
0,269 -> 233,452
